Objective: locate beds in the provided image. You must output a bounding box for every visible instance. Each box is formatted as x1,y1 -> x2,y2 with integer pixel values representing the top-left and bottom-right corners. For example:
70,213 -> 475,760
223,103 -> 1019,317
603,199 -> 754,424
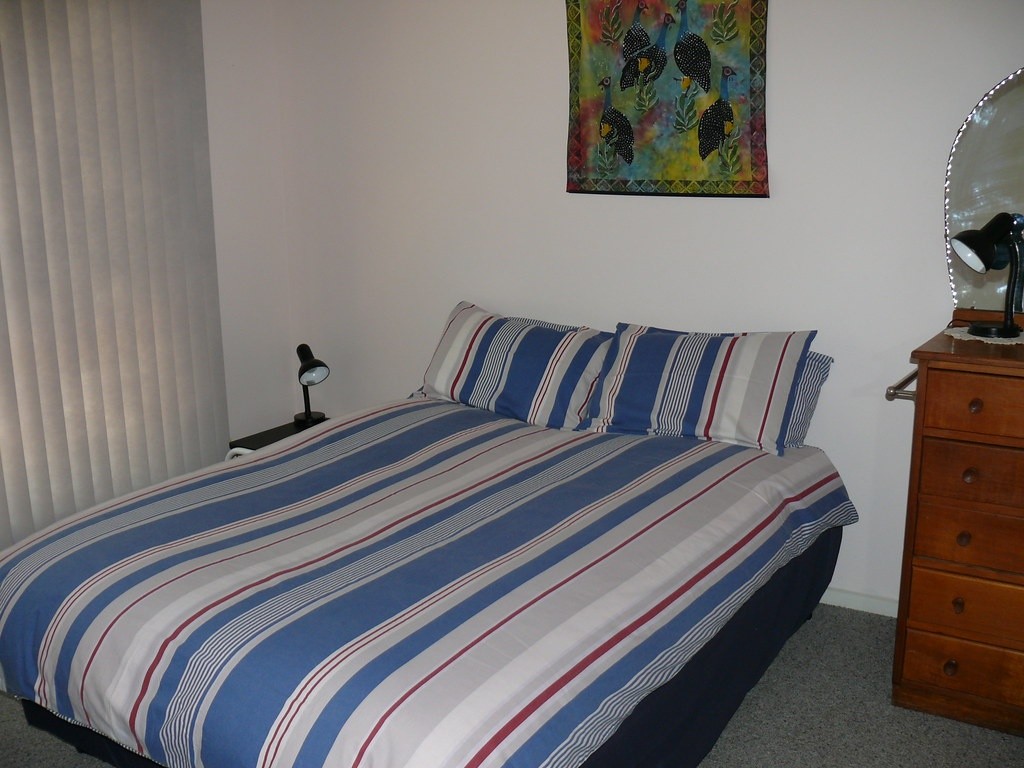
0,382 -> 858,768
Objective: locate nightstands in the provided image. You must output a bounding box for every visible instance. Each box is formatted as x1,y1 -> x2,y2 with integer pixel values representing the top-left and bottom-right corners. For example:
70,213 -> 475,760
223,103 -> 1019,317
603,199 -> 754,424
229,418 -> 330,455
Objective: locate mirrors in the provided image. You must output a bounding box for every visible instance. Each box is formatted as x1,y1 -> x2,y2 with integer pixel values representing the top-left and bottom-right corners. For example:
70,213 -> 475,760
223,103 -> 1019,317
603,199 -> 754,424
943,67 -> 1024,332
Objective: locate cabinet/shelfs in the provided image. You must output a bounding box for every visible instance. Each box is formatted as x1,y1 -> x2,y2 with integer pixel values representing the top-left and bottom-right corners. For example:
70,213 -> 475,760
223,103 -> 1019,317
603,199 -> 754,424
888,307 -> 1024,737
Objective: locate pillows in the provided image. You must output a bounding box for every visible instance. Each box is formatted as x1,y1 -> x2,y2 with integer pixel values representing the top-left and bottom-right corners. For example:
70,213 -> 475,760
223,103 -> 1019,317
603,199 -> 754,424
508,317 -> 579,334
783,352 -> 835,449
575,322 -> 818,456
406,299 -> 614,433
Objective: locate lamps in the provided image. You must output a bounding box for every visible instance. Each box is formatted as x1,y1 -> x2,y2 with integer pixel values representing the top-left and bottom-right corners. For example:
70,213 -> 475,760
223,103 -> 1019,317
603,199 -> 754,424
983,213 -> 1023,313
950,210 -> 1022,339
291,343 -> 330,426
